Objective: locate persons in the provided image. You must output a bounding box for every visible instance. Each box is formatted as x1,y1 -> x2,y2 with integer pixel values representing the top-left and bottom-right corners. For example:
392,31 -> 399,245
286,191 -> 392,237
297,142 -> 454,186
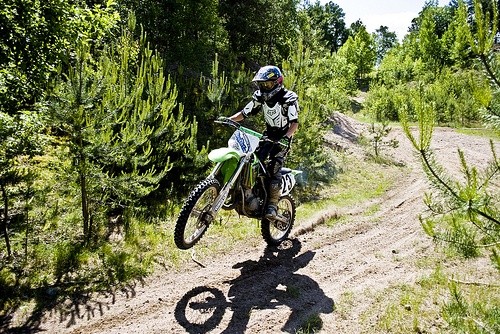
214,64 -> 300,220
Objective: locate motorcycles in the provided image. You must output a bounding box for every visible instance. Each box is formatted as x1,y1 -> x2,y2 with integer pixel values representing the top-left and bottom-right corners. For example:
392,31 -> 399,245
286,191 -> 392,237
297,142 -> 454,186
172,118 -> 304,250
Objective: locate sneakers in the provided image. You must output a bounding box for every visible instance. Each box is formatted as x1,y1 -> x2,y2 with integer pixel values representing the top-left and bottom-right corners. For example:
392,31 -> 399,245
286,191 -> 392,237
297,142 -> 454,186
263,204 -> 279,219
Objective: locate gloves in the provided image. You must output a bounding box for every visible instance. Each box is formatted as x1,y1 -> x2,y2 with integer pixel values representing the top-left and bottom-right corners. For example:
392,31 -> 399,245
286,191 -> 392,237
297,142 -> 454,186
217,115 -> 231,127
273,138 -> 289,151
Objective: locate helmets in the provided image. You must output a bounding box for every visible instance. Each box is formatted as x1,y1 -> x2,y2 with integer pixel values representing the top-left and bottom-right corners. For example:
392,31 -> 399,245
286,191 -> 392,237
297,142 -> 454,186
251,65 -> 284,94
